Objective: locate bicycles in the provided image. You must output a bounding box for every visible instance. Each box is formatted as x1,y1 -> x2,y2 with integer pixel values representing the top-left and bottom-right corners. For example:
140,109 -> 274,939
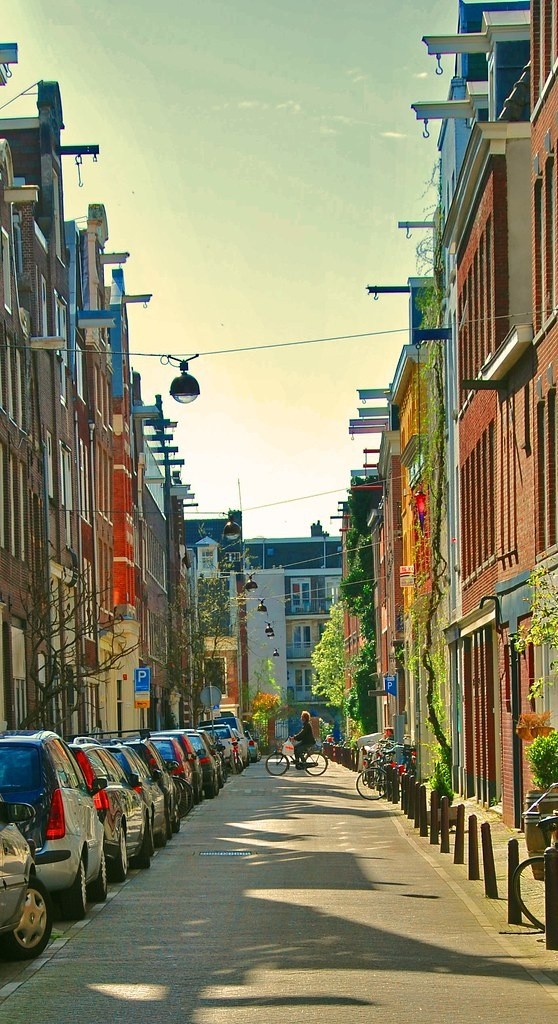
265,735 -> 328,777
512,782 -> 558,932
355,739 -> 416,800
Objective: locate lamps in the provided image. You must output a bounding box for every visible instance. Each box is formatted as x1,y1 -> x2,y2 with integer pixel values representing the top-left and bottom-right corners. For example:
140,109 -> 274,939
243,572 -> 259,593
264,621 -> 275,640
257,598 -> 267,615
160,354 -> 200,403
272,648 -> 280,658
223,514 -> 242,540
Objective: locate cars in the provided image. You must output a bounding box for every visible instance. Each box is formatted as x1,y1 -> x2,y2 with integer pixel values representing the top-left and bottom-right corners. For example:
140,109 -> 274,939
0,723 -> 262,962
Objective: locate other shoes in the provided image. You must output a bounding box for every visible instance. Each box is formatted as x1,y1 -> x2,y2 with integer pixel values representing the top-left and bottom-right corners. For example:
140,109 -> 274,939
291,760 -> 299,763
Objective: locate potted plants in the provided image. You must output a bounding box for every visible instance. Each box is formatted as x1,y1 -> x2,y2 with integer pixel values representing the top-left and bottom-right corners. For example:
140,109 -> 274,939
524,729 -> 558,880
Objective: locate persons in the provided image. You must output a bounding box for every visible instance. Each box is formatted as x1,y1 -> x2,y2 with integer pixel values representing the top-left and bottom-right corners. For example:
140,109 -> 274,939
289,711 -> 316,767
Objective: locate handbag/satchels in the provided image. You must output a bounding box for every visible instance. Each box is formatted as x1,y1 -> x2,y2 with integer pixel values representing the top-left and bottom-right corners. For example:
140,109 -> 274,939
282,737 -> 294,756
